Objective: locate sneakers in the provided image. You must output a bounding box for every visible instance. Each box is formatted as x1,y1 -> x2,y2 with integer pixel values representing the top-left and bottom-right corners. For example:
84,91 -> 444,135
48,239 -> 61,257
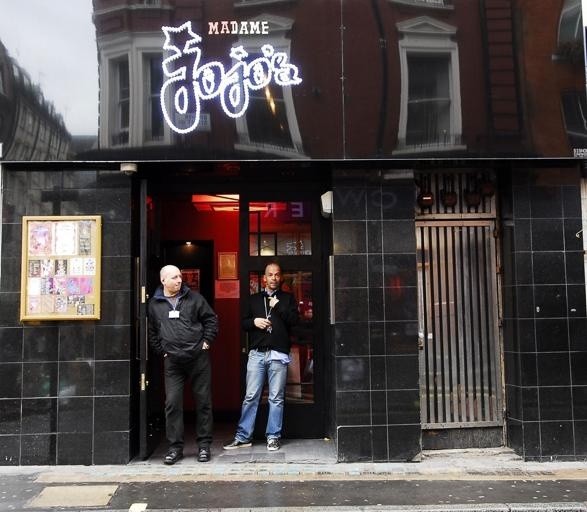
266,439 -> 279,450
222,440 -> 252,450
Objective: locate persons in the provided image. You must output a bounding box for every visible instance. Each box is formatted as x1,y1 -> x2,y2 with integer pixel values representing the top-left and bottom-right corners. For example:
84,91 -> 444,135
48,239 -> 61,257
147,264 -> 221,466
222,259 -> 302,451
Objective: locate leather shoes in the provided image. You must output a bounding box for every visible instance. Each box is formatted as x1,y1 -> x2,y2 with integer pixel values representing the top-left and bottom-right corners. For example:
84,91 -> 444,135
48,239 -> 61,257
163,450 -> 183,465
197,447 -> 210,462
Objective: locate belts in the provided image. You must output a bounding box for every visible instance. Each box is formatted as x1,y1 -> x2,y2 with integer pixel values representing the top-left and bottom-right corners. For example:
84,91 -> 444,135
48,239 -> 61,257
249,346 -> 270,352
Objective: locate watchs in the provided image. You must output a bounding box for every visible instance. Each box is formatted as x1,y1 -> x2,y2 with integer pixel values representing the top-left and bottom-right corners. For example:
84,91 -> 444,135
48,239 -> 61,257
201,342 -> 209,349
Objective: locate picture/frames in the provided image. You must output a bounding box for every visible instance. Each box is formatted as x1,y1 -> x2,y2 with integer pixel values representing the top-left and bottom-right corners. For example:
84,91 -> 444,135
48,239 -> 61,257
19,214 -> 102,324
216,251 -> 238,280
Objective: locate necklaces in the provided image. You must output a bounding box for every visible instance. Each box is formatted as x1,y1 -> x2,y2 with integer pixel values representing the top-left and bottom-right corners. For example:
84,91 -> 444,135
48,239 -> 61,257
263,296 -> 275,334
